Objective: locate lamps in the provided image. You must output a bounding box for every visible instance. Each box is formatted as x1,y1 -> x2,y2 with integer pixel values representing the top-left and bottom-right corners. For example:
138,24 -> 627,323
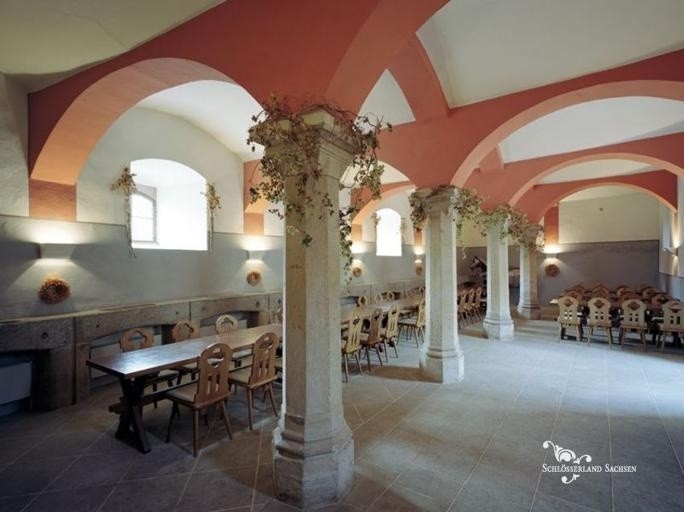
39,243 -> 74,258
248,250 -> 264,261
664,248 -> 676,259
348,253 -> 364,260
543,254 -> 558,259
414,255 -> 425,261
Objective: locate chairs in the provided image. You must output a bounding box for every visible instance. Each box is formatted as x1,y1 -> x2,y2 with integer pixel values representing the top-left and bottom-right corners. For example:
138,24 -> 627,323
557,284 -> 684,353
